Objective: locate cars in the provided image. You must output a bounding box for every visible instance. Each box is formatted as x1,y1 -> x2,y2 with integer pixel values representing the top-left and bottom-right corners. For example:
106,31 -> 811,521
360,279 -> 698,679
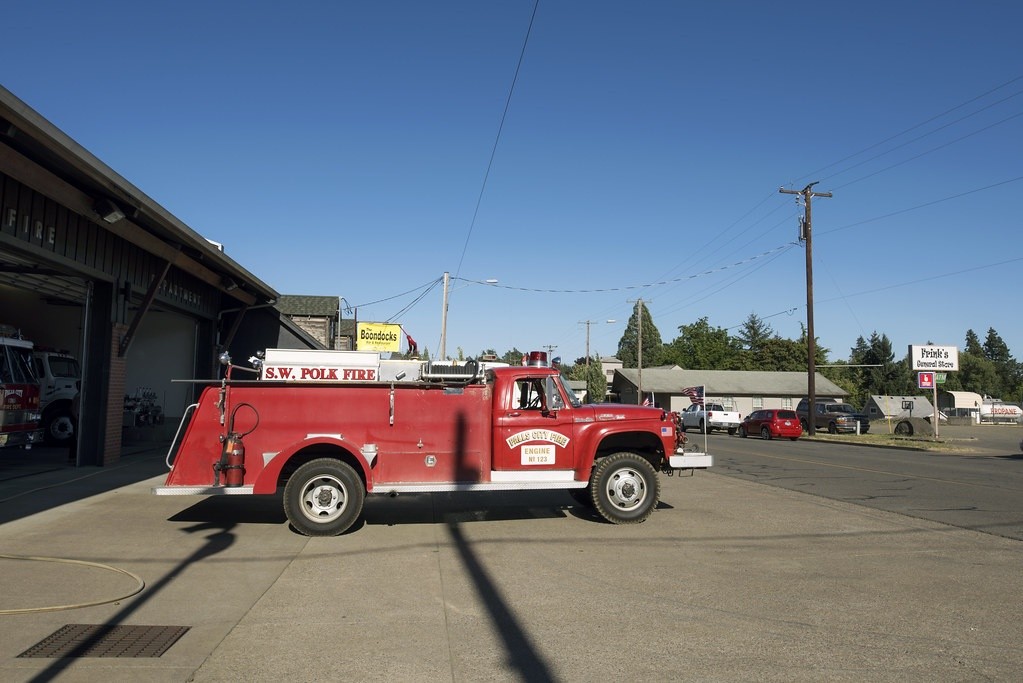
736,409 -> 803,441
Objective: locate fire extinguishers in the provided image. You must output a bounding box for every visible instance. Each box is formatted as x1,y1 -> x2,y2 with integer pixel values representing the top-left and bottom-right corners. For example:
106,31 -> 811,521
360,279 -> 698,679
224,435 -> 244,487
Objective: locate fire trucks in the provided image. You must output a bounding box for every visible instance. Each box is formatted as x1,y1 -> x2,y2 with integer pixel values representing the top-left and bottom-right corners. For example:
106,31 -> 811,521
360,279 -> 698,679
149,344 -> 714,538
1,328 -> 47,469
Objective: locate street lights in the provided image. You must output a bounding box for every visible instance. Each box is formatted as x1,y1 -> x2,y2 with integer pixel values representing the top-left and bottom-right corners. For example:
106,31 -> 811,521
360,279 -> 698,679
440,271 -> 499,362
577,316 -> 618,368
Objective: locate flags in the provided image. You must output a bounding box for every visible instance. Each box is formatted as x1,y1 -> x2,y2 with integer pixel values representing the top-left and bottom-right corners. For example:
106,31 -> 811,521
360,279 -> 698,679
643,395 -> 653,407
682,386 -> 704,407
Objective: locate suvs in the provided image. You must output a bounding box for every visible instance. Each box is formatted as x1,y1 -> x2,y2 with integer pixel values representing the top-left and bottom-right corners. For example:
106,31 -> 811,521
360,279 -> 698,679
796,396 -> 872,435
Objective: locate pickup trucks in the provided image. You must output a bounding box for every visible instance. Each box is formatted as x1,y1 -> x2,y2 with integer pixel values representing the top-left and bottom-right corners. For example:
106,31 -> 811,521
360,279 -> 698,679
680,401 -> 743,435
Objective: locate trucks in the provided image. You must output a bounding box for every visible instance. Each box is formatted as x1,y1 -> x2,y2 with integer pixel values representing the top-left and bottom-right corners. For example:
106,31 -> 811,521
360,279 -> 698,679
34,350 -> 80,447
